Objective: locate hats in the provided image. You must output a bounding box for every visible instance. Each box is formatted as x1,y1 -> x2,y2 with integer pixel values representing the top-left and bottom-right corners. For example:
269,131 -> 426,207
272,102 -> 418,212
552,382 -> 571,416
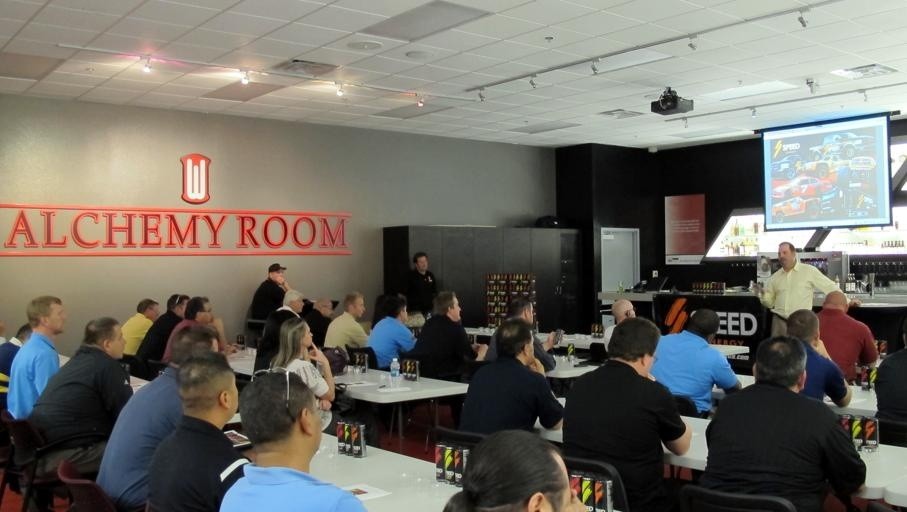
269,263 -> 286,272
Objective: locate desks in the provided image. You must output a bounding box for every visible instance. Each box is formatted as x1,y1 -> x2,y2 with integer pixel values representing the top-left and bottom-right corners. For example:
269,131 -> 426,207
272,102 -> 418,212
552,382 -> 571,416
231,432 -> 469,512
324,362 -> 472,446
223,339 -> 258,381
704,369 -> 879,422
708,344 -> 751,356
53,354 -> 150,395
464,325 -> 605,352
542,352 -> 601,380
523,392 -> 906,512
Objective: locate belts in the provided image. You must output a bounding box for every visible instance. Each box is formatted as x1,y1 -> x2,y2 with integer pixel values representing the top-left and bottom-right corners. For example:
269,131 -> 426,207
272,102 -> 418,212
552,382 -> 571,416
48,437 -> 102,454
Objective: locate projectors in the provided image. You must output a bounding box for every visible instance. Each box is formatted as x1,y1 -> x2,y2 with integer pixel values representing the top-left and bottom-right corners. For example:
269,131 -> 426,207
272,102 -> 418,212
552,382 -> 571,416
650,96 -> 694,116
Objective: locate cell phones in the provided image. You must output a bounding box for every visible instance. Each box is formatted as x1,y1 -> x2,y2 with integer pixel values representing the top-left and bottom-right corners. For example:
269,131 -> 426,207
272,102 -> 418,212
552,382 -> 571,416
552,328 -> 567,348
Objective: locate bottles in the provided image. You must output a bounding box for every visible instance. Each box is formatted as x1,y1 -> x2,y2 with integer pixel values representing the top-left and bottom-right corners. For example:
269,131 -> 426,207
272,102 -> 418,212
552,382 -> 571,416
846,272 -> 856,293
390,358 -> 401,388
835,273 -> 840,285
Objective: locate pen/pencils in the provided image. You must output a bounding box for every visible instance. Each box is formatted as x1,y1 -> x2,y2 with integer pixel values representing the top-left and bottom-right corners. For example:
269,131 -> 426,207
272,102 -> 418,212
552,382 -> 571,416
379,385 -> 385,388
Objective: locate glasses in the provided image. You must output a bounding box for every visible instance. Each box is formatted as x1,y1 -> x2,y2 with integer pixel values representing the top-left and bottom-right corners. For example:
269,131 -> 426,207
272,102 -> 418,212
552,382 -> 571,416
251,367 -> 295,422
149,306 -> 162,316
173,294 -> 180,305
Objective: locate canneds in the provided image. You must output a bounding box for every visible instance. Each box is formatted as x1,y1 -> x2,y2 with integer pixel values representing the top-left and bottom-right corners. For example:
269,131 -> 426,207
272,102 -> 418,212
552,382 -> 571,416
433,441 -> 471,490
485,271 -> 534,329
859,365 -> 877,390
400,360 -> 419,381
841,414 -> 879,452
338,421 -> 366,458
353,352 -> 368,373
590,324 -> 604,336
880,339 -> 887,357
567,468 -> 614,511
692,282 -> 725,295
568,343 -> 574,357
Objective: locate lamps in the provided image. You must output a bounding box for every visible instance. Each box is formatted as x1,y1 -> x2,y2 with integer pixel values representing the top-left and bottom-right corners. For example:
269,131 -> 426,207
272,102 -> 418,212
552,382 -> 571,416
240,69 -> 250,84
337,84 -> 345,96
143,57 -> 153,72
417,97 -> 425,107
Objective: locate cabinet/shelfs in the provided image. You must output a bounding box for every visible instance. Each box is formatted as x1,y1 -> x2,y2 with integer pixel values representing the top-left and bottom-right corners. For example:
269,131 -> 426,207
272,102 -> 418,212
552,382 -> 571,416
442,226 -> 475,326
475,227 -> 503,325
531,228 -> 561,325
503,227 -> 531,273
383,226 -> 443,293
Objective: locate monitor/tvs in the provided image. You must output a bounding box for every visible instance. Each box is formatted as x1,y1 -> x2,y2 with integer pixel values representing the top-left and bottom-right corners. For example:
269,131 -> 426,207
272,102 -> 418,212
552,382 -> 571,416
643,275 -> 668,291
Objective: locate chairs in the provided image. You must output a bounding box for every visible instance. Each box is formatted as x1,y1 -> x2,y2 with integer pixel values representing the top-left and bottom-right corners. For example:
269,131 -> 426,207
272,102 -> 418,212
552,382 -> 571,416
122,353 -> 144,377
675,480 -> 795,511
56,459 -> 142,512
0,410 -> 104,511
344,344 -> 379,439
388,351 -> 441,443
561,455 -> 631,512
431,424 -> 492,451
589,342 -> 609,362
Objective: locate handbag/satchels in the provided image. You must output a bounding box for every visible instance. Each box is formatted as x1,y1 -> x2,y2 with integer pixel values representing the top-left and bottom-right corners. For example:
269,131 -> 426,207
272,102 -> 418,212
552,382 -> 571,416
310,346 -> 348,376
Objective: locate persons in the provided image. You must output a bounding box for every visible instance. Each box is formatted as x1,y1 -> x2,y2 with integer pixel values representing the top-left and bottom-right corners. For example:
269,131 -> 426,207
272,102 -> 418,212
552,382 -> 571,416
322,290 -> 369,366
484,293 -> 560,372
815,290 -> 879,382
410,289 -> 490,384
219,367 -> 370,512
132,295 -> 192,375
442,429 -> 590,512
749,240 -> 861,338
304,296 -> 335,349
602,299 -> 636,358
562,318 -> 691,512
248,263 -> 293,330
269,318 -> 380,447
697,335 -> 866,512
874,309 -> 907,445
0,324 -> 33,447
783,310 -> 852,407
403,251 -> 437,316
650,308 -> 743,418
119,298 -> 159,373
5,295 -> 70,512
15,315 -> 134,478
158,295 -> 236,370
365,293 -> 417,373
94,323 -> 220,512
260,289 -> 305,346
459,318 -> 564,436
145,351 -> 250,512
